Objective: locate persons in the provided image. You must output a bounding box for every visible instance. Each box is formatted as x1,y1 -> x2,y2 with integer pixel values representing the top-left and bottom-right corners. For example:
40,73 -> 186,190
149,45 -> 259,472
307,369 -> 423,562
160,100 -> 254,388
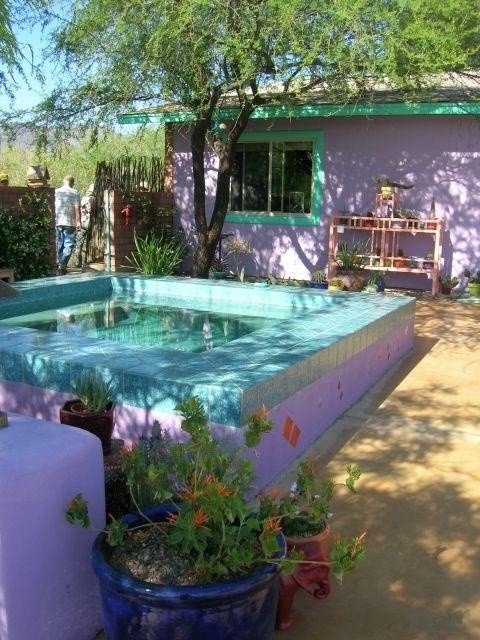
55,175 -> 81,275
75,182 -> 95,268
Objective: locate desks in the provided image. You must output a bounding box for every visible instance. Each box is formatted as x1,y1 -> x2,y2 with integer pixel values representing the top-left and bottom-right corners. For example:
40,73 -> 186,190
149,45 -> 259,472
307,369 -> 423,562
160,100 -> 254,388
0,410 -> 109,639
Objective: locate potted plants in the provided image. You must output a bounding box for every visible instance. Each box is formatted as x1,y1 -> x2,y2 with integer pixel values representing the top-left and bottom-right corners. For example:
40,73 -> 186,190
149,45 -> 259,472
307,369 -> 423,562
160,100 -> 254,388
0,174 -> 9,186
60,372 -> 117,455
208,258 -> 228,279
279,511 -> 330,557
308,177 -> 480,296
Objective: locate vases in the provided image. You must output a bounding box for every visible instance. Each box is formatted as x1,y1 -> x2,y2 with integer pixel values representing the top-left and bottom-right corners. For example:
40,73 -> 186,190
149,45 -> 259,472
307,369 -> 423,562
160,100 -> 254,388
91,502 -> 285,640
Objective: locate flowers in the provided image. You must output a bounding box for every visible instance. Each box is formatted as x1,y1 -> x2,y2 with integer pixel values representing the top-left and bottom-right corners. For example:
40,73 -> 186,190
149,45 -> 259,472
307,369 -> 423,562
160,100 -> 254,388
64,394 -> 366,586
